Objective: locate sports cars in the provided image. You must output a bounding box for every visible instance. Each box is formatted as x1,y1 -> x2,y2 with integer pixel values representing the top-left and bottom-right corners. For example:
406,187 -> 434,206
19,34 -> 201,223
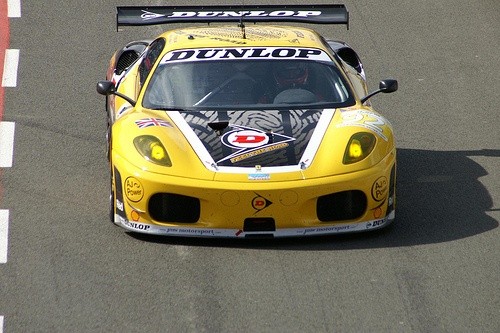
96,4 -> 399,239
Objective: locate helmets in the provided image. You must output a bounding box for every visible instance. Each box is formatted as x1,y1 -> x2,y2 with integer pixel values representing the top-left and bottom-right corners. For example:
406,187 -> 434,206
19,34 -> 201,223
272,59 -> 309,90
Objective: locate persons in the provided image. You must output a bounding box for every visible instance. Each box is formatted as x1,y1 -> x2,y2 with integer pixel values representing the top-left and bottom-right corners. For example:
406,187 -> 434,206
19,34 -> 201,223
256,59 -> 324,105
